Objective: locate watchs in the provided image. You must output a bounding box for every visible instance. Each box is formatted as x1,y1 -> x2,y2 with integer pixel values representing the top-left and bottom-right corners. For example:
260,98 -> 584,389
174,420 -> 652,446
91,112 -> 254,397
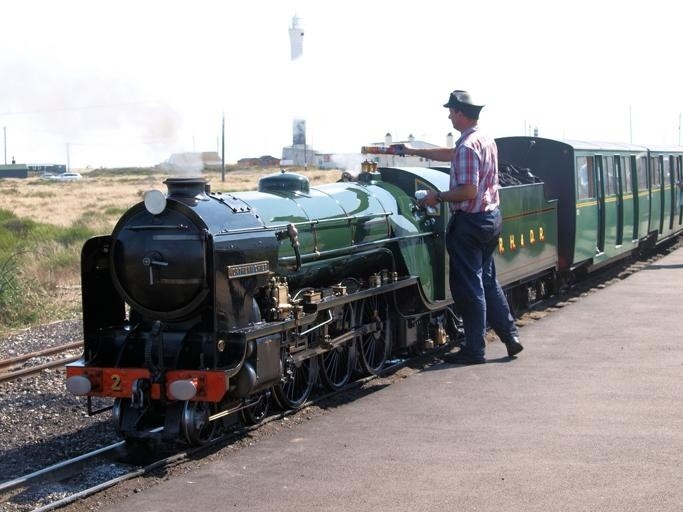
434,191 -> 444,204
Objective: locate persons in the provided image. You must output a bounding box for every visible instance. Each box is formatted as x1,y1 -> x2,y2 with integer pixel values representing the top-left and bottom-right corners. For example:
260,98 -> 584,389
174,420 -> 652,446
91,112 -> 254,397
390,90 -> 524,365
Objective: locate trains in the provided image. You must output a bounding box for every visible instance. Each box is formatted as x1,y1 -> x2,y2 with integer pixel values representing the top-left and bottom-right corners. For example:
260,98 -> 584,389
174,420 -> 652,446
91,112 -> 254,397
67,134 -> 682,448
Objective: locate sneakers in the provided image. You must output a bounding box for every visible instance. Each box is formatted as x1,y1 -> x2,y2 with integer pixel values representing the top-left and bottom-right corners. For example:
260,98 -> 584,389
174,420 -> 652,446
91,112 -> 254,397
449,348 -> 486,364
507,337 -> 524,356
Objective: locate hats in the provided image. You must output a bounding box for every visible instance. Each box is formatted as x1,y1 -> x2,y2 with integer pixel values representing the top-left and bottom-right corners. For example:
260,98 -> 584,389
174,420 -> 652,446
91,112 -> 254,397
443,90 -> 486,108
